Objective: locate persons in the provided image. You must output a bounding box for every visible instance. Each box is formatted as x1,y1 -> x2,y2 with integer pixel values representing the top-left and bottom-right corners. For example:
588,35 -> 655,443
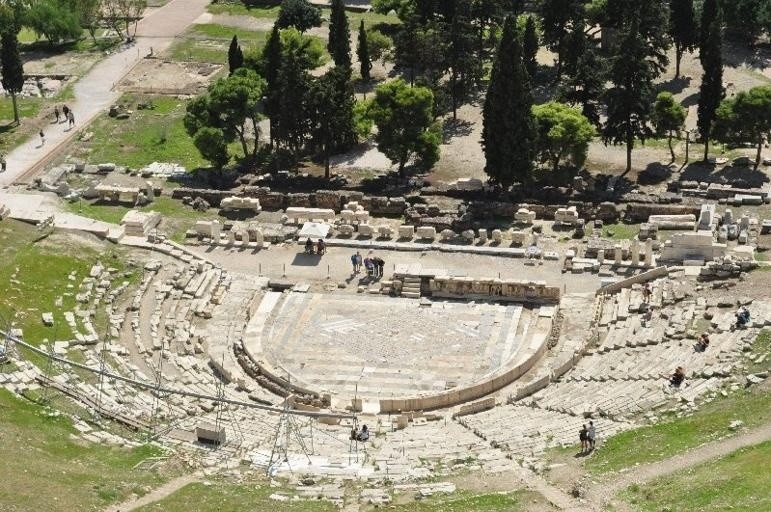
316,239 -> 327,255
697,338 -> 707,348
305,237 -> 313,255
350,428 -> 360,440
668,369 -> 680,388
39,128 -> 47,145
741,308 -> 750,319
53,105 -> 59,122
672,366 -> 684,381
63,104 -> 68,119
358,425 -> 368,440
578,424 -> 589,453
642,278 -> 652,303
350,250 -> 385,278
0,154 -> 7,170
730,313 -> 746,330
701,335 -> 709,344
67,108 -> 74,126
587,421 -> 595,450
